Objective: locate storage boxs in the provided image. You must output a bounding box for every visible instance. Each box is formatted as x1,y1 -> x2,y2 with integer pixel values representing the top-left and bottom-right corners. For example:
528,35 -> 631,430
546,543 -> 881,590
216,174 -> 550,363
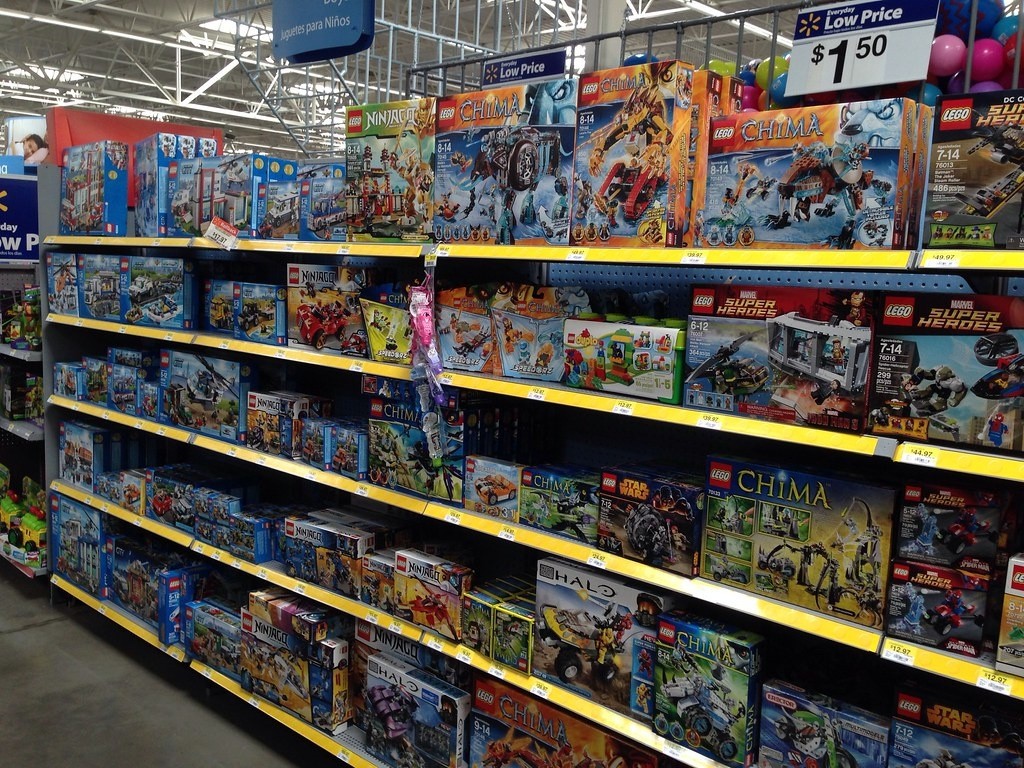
51,57 -> 1024,767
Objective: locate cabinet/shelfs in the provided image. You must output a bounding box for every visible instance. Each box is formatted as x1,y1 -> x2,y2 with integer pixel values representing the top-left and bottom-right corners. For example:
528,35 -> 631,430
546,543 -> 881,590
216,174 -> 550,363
0,343 -> 45,579
46,234 -> 438,768
435,243 -> 1022,766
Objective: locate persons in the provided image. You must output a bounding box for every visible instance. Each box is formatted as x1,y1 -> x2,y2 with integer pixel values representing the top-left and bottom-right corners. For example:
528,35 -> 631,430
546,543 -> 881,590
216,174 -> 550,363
6,128 -> 51,164
